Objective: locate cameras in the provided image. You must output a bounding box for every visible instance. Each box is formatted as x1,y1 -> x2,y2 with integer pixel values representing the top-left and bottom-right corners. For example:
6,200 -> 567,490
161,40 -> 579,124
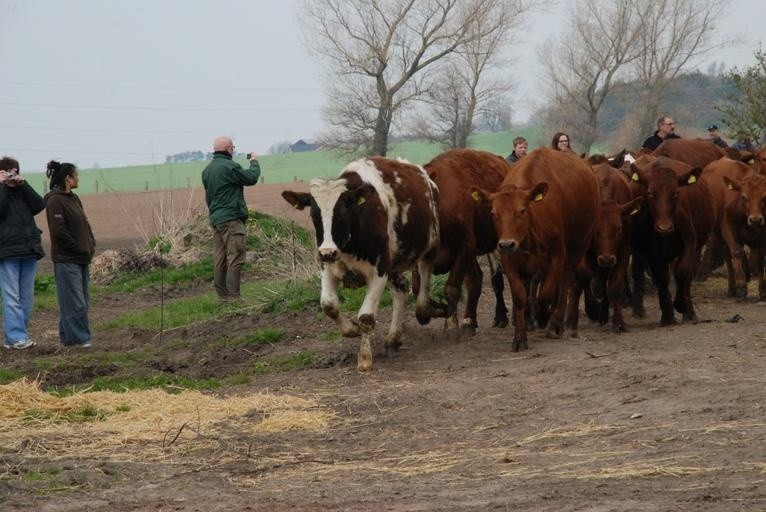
247,154 -> 251,159
3,172 -> 16,181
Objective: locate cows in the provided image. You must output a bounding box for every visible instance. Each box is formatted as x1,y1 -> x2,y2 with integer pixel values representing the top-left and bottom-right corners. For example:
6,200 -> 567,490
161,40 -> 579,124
281,155 -> 441,372
413,136 -> 766,352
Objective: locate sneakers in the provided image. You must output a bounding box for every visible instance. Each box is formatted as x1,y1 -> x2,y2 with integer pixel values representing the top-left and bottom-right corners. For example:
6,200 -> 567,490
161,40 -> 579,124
4,338 -> 36,350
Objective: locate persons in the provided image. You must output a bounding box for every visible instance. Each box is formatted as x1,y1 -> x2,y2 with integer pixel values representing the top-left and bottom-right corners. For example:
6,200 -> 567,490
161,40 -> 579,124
0,155 -> 45,351
730,129 -> 756,152
706,124 -> 728,148
43,160 -> 96,347
199,136 -> 261,302
551,132 -> 570,152
641,115 -> 681,152
504,136 -> 528,166
